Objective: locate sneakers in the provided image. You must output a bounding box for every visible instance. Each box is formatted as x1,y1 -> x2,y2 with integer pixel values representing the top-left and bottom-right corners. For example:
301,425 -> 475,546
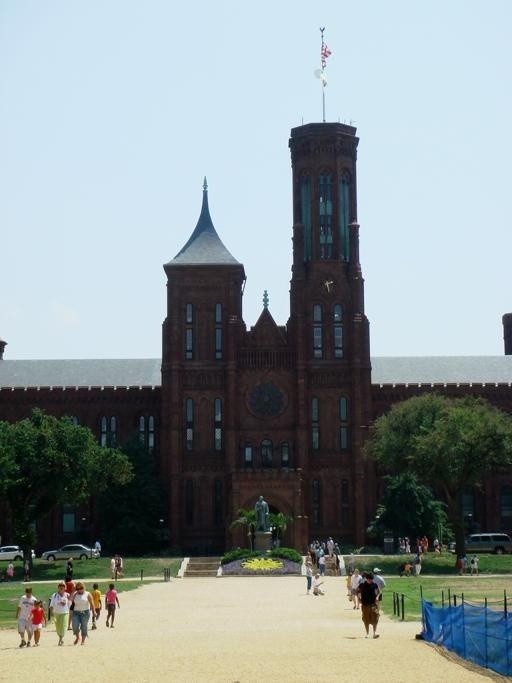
19,640 -> 26,647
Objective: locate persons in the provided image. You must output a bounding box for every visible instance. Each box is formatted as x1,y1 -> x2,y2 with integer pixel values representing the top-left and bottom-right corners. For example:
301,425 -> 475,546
6,561 -> 14,581
104,584 -> 120,628
398,535 -> 442,579
462,550 -> 467,566
66,557 -> 74,578
470,554 -> 480,574
108,554 -> 125,579
305,535 -> 386,638
455,554 -> 465,575
24,560 -> 30,581
255,495 -> 269,531
95,537 -> 102,552
15,576 -> 102,649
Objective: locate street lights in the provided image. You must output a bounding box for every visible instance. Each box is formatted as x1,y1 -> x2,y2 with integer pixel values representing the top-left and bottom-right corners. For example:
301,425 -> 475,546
159,517 -> 166,555
467,511 -> 472,529
376,514 -> 380,530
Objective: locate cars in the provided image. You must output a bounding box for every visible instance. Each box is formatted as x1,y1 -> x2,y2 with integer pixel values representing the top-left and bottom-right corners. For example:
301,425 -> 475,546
41,543 -> 100,560
0,545 -> 36,561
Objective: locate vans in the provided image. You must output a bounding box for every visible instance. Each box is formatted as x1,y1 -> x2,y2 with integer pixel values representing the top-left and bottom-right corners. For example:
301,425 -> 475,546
446,532 -> 512,554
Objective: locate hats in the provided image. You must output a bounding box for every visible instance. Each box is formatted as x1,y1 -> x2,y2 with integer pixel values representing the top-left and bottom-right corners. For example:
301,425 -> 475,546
373,568 -> 381,572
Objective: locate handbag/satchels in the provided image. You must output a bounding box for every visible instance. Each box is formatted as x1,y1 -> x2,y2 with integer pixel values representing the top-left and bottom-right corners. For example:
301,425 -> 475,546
70,602 -> 74,610
